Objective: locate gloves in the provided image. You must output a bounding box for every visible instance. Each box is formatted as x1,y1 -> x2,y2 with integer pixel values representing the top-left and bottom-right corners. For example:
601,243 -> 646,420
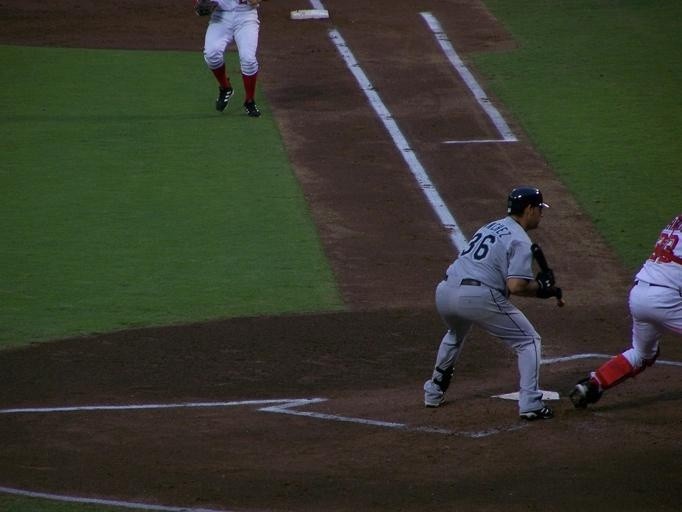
536,269 -> 553,290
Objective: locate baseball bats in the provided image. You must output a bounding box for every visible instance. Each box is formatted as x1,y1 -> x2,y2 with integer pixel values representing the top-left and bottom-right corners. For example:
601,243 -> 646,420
530,243 -> 568,305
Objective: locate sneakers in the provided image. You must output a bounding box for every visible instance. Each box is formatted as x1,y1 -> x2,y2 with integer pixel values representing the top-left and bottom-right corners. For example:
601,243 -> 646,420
244,102 -> 261,117
567,376 -> 602,408
519,406 -> 553,420
425,395 -> 446,408
216,86 -> 234,112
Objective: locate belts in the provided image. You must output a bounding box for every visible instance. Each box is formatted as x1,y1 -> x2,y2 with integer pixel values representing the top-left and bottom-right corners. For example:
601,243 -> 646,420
635,281 -> 669,288
444,275 -> 481,286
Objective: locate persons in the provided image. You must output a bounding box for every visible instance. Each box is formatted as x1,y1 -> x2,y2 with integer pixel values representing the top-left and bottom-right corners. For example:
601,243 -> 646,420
425,186 -> 562,420
194,0 -> 264,117
571,214 -> 681,410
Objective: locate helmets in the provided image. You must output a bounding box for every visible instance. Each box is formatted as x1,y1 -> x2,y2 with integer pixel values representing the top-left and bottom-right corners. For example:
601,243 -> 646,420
508,186 -> 551,214
